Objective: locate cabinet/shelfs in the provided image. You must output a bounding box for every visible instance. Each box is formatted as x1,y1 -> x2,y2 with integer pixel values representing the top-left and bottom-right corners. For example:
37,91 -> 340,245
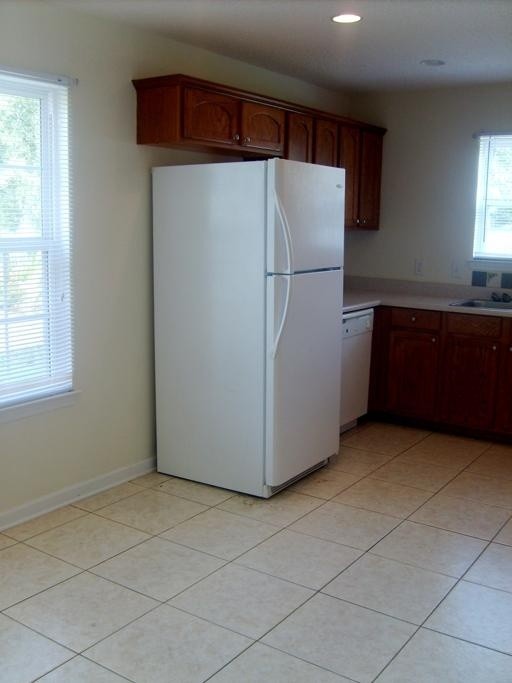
366,304 -> 442,434
282,99 -> 339,166
130,73 -> 284,156
337,115 -> 387,230
440,311 -> 512,447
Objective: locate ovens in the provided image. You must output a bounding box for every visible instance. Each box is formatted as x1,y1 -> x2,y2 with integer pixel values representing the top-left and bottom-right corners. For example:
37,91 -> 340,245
335,315 -> 374,430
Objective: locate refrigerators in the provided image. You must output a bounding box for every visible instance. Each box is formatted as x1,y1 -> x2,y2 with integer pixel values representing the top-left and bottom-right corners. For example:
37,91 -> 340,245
148,156 -> 346,499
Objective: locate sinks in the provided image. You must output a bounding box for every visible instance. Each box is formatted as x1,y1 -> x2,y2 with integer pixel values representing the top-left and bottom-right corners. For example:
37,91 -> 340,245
448,297 -> 512,309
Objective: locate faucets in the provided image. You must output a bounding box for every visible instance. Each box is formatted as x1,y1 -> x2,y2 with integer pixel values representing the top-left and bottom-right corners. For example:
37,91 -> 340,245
490,292 -> 499,302
